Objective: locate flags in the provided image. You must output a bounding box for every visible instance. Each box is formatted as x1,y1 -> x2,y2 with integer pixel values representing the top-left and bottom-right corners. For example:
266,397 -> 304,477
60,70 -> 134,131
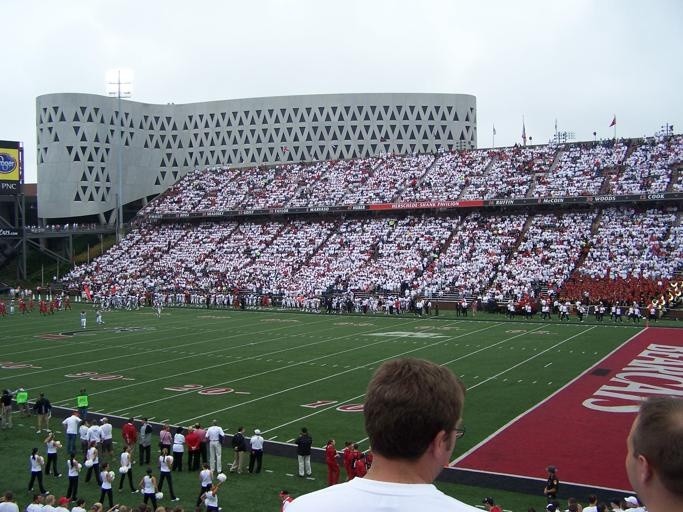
493,127 -> 497,135
521,123 -> 526,140
609,118 -> 616,127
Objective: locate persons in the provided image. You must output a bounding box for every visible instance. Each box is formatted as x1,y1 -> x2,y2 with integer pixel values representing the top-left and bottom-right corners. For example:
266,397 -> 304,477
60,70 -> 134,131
527,494 -> 646,512
1,384 -> 373,512
544,465 -> 560,505
621,395 -> 683,511
1,131 -> 683,329
481,497 -> 502,512
282,357 -> 485,511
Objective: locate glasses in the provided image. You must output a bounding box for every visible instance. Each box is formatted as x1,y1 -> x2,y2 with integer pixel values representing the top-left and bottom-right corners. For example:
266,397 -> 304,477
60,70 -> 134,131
442,422 -> 465,439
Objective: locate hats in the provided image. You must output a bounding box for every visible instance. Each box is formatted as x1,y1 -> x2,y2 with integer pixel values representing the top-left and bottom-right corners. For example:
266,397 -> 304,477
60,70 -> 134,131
278,489 -> 288,496
254,429 -> 261,435
623,495 -> 638,505
57,496 -> 70,504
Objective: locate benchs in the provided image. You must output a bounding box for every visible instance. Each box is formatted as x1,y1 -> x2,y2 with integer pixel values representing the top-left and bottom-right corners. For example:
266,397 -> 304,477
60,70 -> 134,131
30,132 -> 682,325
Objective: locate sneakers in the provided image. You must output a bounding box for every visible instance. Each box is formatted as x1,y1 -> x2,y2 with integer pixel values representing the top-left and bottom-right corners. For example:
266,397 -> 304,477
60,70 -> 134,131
37,429 -> 52,435
217,507 -> 223,511
28,460 -> 181,502
